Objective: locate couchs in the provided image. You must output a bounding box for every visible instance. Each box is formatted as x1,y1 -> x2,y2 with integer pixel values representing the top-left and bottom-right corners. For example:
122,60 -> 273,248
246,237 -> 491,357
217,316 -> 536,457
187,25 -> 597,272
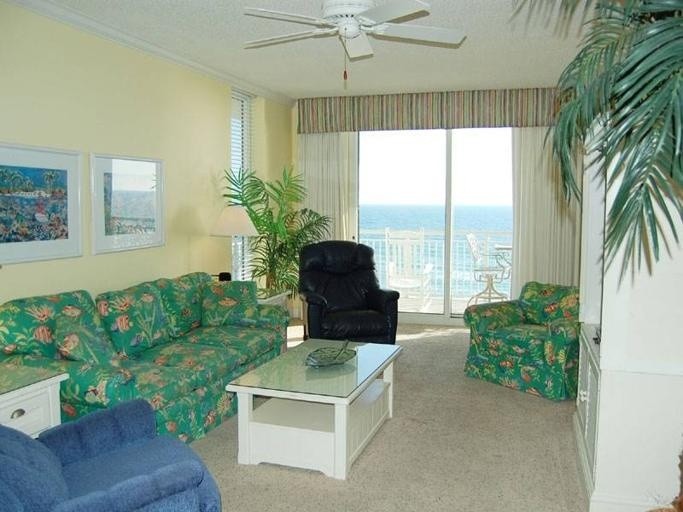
1,272 -> 290,445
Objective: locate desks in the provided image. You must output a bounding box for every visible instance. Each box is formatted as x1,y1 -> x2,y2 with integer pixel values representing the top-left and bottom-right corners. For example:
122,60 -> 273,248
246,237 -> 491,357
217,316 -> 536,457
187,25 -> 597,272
1,363 -> 70,438
494,244 -> 514,279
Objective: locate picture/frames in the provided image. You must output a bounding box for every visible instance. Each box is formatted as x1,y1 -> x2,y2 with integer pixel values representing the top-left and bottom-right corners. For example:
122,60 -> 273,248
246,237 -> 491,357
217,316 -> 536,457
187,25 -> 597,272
0,141 -> 83,265
89,151 -> 166,255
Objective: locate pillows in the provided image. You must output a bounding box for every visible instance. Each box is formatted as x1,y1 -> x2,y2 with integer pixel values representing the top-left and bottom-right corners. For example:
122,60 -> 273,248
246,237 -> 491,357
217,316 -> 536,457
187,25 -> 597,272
200,280 -> 260,328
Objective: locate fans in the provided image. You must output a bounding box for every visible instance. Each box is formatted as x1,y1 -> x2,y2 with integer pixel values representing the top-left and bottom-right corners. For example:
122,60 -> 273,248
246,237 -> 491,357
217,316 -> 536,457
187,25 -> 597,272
244,0 -> 467,60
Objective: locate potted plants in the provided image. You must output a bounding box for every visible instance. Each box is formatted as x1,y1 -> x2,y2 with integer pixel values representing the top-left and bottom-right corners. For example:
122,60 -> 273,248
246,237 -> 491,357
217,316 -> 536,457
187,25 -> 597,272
219,165 -> 334,322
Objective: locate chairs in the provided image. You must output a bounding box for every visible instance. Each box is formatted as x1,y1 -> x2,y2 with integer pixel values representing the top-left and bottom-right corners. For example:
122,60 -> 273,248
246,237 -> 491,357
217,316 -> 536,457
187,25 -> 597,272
386,227 -> 435,313
298,240 -> 399,344
466,233 -> 509,308
463,281 -> 580,401
0,399 -> 222,512
